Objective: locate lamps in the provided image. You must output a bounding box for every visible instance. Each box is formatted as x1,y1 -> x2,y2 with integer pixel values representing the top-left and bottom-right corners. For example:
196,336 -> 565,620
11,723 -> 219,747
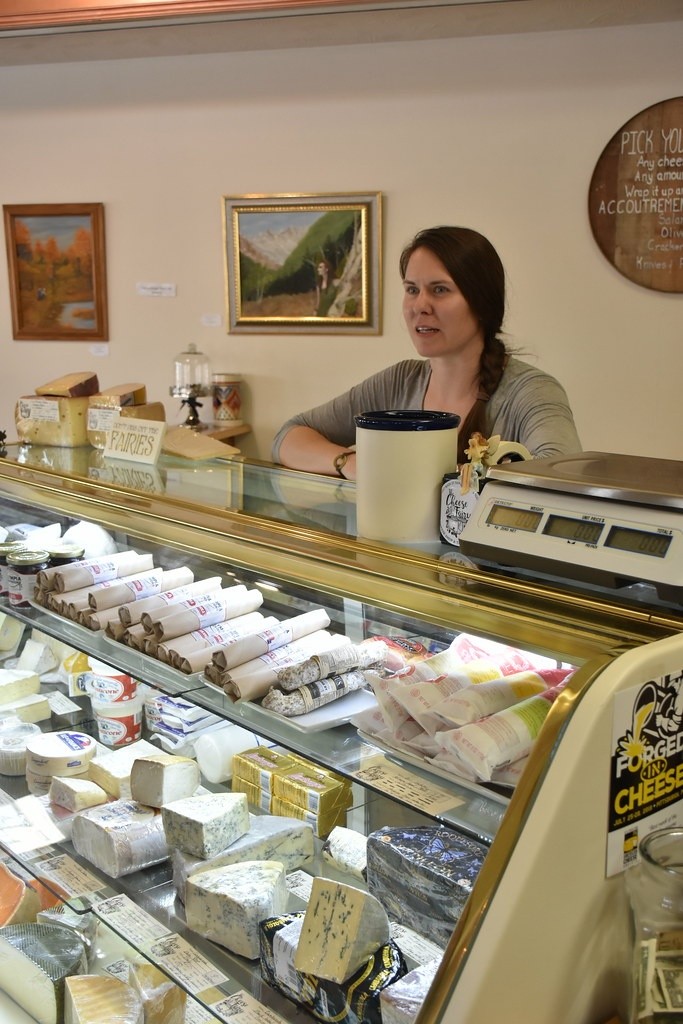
168,343 -> 213,433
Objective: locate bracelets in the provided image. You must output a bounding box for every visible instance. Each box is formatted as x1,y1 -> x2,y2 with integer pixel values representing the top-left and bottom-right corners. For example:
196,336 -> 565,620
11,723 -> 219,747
334,451 -> 356,479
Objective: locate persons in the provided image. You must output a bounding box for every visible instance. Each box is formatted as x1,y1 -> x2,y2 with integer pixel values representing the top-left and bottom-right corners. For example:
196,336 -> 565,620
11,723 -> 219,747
273,225 -> 582,480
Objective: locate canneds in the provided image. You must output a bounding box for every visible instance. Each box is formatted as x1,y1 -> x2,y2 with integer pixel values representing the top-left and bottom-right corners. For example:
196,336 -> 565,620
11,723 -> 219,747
0,542 -> 86,609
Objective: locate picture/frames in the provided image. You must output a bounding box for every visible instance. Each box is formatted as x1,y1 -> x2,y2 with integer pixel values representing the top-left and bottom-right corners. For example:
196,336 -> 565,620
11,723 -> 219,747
2,203 -> 111,342
223,190 -> 384,336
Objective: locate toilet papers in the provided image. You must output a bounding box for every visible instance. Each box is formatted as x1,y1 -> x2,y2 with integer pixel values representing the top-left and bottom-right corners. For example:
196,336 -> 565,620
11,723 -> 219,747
483,439 -> 533,464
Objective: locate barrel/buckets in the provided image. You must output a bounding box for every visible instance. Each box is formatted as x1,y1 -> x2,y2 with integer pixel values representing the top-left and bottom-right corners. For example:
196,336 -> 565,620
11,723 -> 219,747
353,411 -> 461,544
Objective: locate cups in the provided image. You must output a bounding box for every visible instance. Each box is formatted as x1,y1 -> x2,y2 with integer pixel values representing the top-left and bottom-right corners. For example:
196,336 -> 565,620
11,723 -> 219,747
626,826 -> 683,1024
211,374 -> 244,425
441,472 -> 481,545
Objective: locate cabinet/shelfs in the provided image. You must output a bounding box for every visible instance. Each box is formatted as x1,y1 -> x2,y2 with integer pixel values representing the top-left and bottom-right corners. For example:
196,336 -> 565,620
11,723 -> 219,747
0,442 -> 683,1024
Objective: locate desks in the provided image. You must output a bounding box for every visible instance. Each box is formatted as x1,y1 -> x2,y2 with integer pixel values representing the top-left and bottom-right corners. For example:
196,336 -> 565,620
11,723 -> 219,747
193,422 -> 251,443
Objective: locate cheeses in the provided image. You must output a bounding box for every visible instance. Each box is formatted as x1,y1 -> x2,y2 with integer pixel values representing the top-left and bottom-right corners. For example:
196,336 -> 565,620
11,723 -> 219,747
16,372 -> 164,450
1,640 -> 389,1023
